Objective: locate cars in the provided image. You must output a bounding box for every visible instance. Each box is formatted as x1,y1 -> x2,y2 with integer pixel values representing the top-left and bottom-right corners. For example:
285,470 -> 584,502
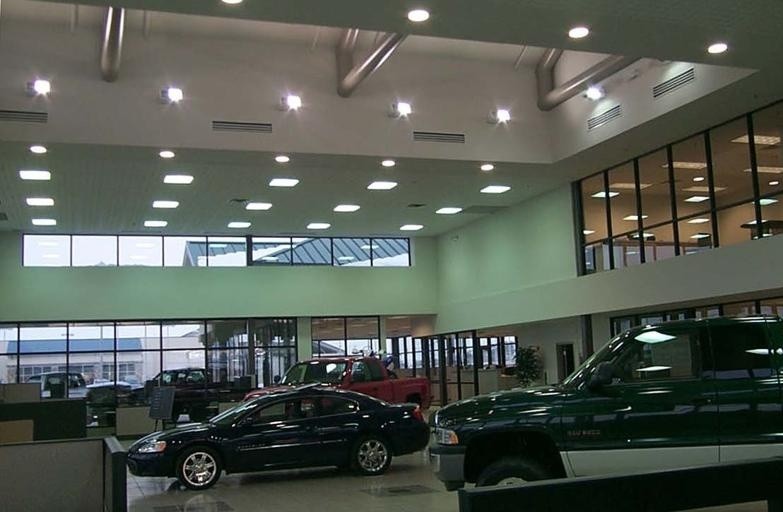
127,382 -> 430,490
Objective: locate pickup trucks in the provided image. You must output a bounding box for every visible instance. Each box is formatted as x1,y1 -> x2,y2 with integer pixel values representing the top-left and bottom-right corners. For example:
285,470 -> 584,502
245,355 -> 431,411
24,367 -> 237,423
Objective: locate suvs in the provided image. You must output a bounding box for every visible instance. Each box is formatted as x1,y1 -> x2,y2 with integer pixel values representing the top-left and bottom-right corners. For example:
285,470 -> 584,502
431,313 -> 781,509
